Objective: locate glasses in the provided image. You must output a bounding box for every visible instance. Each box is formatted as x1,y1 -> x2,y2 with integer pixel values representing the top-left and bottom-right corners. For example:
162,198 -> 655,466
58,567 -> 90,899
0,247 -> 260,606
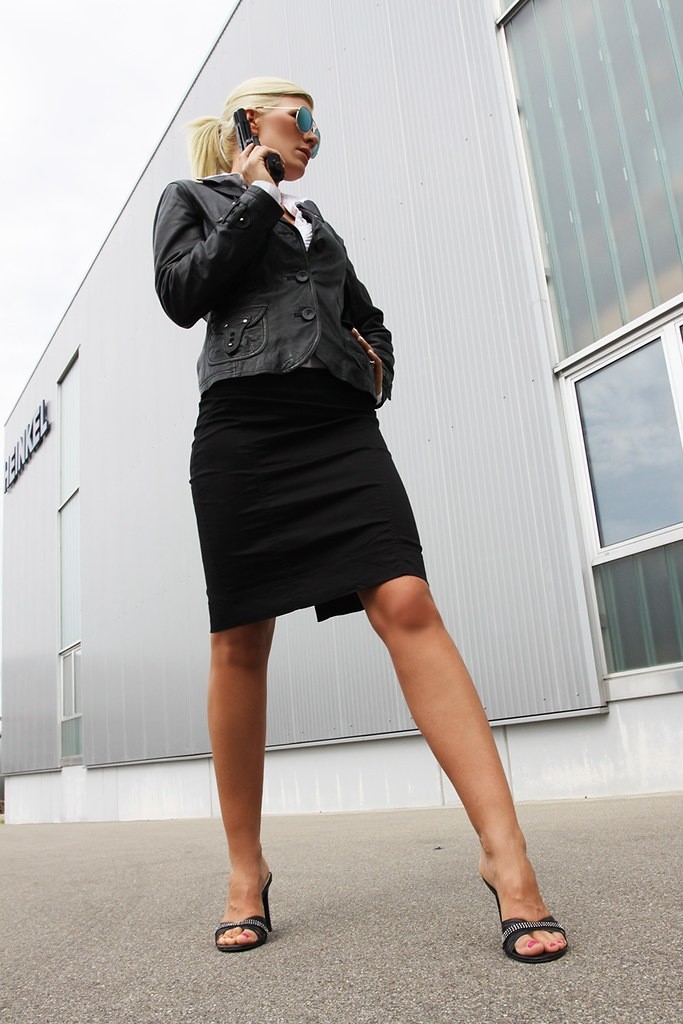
252,105 -> 321,159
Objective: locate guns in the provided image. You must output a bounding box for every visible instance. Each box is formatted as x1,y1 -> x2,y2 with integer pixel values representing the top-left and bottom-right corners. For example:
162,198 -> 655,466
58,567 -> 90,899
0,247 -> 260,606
231,106 -> 285,186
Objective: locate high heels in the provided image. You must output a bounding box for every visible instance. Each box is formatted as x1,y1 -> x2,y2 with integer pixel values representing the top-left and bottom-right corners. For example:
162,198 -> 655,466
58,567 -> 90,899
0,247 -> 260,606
481,876 -> 569,964
214,871 -> 272,953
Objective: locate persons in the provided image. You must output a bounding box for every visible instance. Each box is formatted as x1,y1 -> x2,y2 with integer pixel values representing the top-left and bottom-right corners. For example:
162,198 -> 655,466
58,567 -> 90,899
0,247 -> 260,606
154,76 -> 568,965
